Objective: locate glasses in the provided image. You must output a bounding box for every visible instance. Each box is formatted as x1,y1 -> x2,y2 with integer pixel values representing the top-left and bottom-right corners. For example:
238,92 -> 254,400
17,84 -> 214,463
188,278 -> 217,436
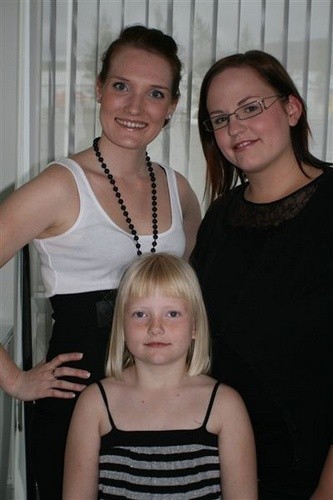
202,94 -> 284,133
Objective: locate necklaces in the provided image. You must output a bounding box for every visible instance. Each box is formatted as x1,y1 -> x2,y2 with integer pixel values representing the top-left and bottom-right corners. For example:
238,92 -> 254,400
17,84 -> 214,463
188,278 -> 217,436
93,137 -> 158,256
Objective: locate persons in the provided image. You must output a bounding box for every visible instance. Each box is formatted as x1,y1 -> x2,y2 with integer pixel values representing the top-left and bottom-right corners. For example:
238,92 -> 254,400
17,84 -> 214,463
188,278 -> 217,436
0,25 -> 203,500
188,51 -> 333,500
63,252 -> 257,500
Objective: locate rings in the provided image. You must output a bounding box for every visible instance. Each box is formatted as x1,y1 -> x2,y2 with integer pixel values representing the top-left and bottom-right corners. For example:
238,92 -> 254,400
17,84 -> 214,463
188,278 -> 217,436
52,369 -> 56,376
